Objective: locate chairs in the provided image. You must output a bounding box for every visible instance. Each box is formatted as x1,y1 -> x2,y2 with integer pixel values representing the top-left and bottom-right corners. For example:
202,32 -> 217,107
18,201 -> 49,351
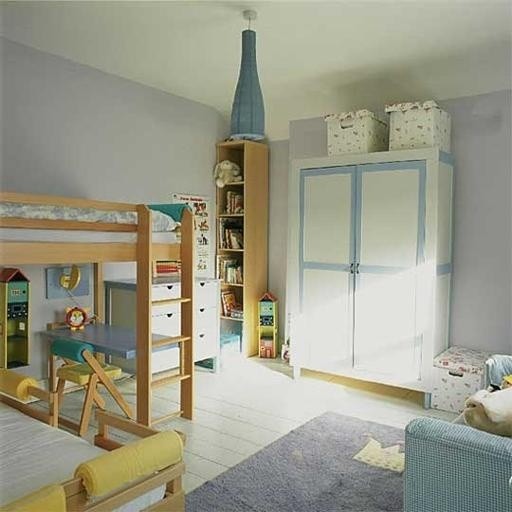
50,338 -> 133,437
404,355 -> 512,512
0,365 -> 185,512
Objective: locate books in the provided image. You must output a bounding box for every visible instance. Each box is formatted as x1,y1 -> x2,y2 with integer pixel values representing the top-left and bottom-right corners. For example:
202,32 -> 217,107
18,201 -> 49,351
215,191 -> 245,320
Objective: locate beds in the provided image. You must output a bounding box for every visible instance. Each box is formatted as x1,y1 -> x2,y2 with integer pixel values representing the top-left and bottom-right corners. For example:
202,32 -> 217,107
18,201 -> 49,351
0,191 -> 194,426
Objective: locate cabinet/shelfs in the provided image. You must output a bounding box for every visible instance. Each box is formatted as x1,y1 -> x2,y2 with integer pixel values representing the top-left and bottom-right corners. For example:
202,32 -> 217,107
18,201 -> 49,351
258,290 -> 279,358
0,268 -> 30,372
105,275 -> 218,382
289,146 -> 454,409
214,140 -> 268,358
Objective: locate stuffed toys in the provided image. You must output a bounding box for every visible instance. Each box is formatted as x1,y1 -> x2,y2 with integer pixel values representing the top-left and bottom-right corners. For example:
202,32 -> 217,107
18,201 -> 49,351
213,159 -> 243,188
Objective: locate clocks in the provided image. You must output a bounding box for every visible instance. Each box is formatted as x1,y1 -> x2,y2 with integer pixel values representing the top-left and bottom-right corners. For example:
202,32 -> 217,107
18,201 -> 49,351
65,307 -> 89,332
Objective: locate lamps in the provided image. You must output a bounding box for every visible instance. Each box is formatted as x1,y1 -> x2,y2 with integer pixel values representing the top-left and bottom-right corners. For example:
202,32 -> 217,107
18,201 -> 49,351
231,12 -> 265,141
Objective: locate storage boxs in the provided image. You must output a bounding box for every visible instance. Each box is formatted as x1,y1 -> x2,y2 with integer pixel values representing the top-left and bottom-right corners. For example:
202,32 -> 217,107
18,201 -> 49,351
385,100 -> 451,151
220,334 -> 240,368
430,343 -> 500,415
324,109 -> 389,156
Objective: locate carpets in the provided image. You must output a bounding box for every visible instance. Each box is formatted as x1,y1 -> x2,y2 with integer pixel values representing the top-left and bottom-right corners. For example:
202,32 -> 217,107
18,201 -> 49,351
183,411 -> 404,512
